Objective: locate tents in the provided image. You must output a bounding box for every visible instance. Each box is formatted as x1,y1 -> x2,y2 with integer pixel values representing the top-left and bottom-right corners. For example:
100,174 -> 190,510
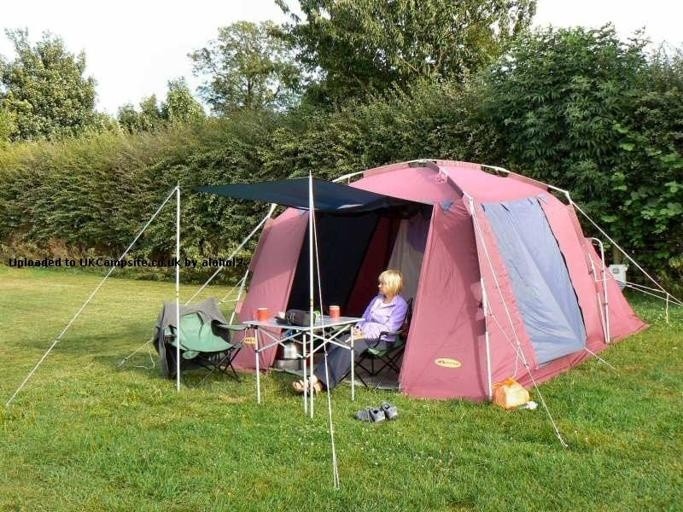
179,159 -> 653,402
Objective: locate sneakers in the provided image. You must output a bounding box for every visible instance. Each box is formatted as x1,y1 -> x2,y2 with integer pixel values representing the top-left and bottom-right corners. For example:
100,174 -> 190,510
355,406 -> 385,423
381,401 -> 398,421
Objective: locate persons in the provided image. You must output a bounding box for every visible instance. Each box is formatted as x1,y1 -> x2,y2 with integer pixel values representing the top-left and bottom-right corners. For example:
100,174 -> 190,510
293,270 -> 408,396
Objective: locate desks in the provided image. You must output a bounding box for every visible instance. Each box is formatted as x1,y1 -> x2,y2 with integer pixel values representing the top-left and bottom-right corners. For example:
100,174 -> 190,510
242,313 -> 365,404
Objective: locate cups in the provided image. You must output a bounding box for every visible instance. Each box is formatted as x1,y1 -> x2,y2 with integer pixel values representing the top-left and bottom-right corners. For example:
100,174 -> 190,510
255,308 -> 269,323
329,305 -> 341,318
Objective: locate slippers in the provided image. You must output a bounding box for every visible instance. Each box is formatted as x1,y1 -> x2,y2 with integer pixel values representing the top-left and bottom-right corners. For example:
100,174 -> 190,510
306,384 -> 321,395
292,377 -> 311,391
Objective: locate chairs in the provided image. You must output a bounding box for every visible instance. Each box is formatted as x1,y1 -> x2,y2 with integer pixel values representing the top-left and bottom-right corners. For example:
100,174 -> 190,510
158,297 -> 254,391
337,297 -> 413,391
